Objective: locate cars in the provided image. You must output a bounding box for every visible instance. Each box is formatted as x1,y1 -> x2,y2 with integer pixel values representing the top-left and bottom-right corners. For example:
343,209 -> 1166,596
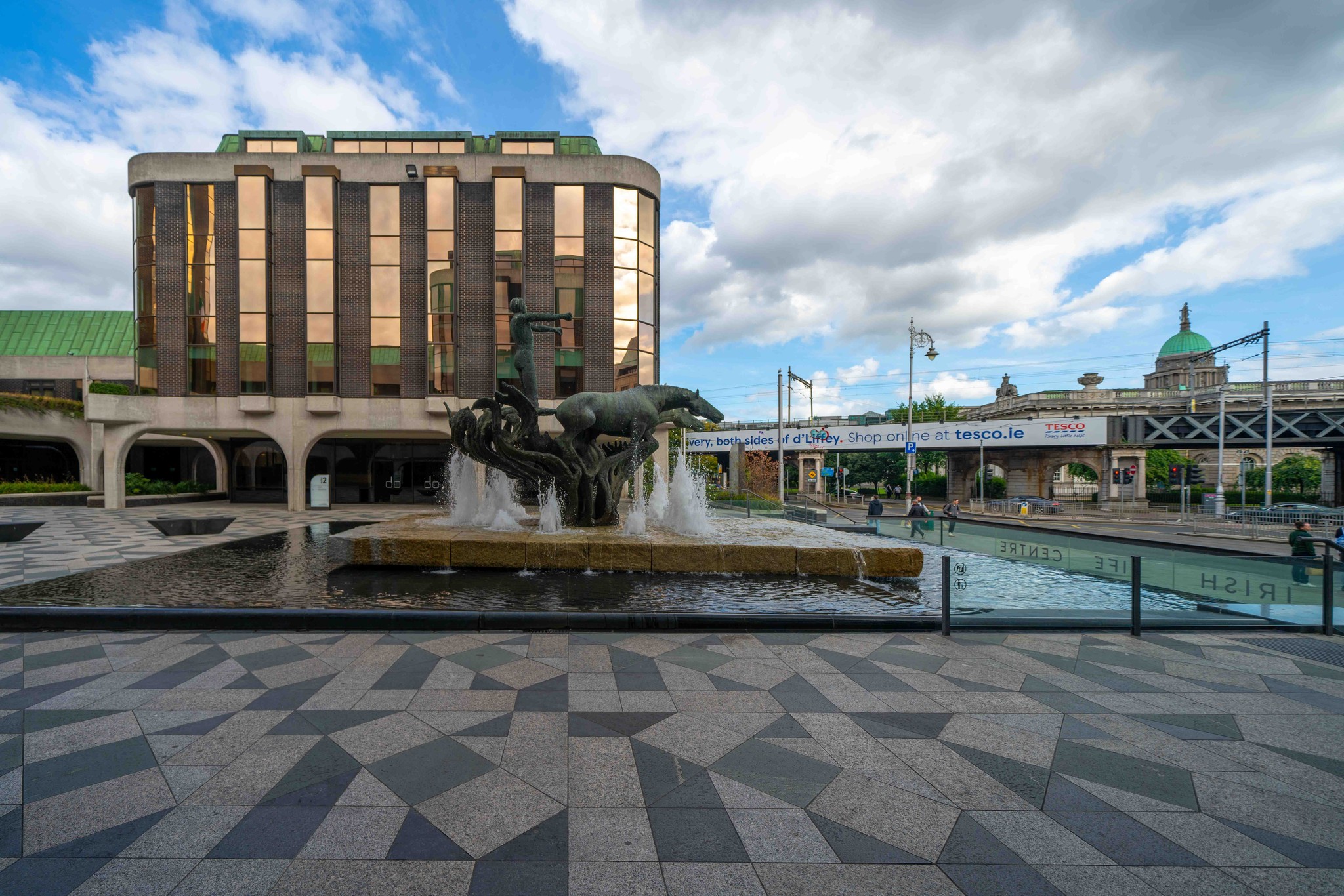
1225,502 -> 1344,527
985,496 -> 1065,515
824,489 -> 865,503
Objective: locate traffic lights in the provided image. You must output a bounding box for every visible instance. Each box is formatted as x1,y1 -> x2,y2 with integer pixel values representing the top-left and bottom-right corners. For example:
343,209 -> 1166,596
1169,464 -> 1180,485
984,466 -> 993,483
1123,468 -> 1133,485
1187,464 -> 1205,485
838,467 -> 843,480
1113,468 -> 1121,484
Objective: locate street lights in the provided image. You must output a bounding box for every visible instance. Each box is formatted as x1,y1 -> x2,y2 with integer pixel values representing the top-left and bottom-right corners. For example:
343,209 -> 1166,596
905,317 -> 940,516
1237,450 -> 1251,534
1214,369 -> 1235,519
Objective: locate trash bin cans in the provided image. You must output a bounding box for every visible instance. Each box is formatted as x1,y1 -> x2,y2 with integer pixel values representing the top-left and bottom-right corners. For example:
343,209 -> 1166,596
920,510 -> 934,531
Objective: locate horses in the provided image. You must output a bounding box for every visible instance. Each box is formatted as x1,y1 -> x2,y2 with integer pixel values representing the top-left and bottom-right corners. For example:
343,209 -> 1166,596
534,384 -> 724,475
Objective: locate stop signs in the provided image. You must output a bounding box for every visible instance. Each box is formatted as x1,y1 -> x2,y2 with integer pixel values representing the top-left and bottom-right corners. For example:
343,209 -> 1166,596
1130,464 -> 1136,474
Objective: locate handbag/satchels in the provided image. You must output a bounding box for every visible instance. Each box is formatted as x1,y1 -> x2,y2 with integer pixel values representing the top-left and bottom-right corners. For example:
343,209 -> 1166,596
905,520 -> 911,529
886,486 -> 891,493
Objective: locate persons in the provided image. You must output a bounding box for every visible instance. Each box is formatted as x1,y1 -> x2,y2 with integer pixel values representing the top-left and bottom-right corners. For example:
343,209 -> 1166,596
886,484 -> 892,501
1334,527 -> 1344,591
943,499 -> 960,537
1289,521 -> 1316,587
868,495 -> 883,532
908,496 -> 930,539
509,298 -> 574,434
894,485 -> 902,500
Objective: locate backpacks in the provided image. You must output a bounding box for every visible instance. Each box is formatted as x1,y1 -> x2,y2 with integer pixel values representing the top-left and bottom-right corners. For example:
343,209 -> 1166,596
943,504 -> 958,515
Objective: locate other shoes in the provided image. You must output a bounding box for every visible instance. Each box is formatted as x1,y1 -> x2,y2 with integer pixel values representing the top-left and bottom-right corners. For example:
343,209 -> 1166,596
910,536 -> 915,538
948,532 -> 955,537
1292,581 -> 1301,586
1299,583 -> 1316,587
922,533 -> 926,539
887,498 -> 889,501
